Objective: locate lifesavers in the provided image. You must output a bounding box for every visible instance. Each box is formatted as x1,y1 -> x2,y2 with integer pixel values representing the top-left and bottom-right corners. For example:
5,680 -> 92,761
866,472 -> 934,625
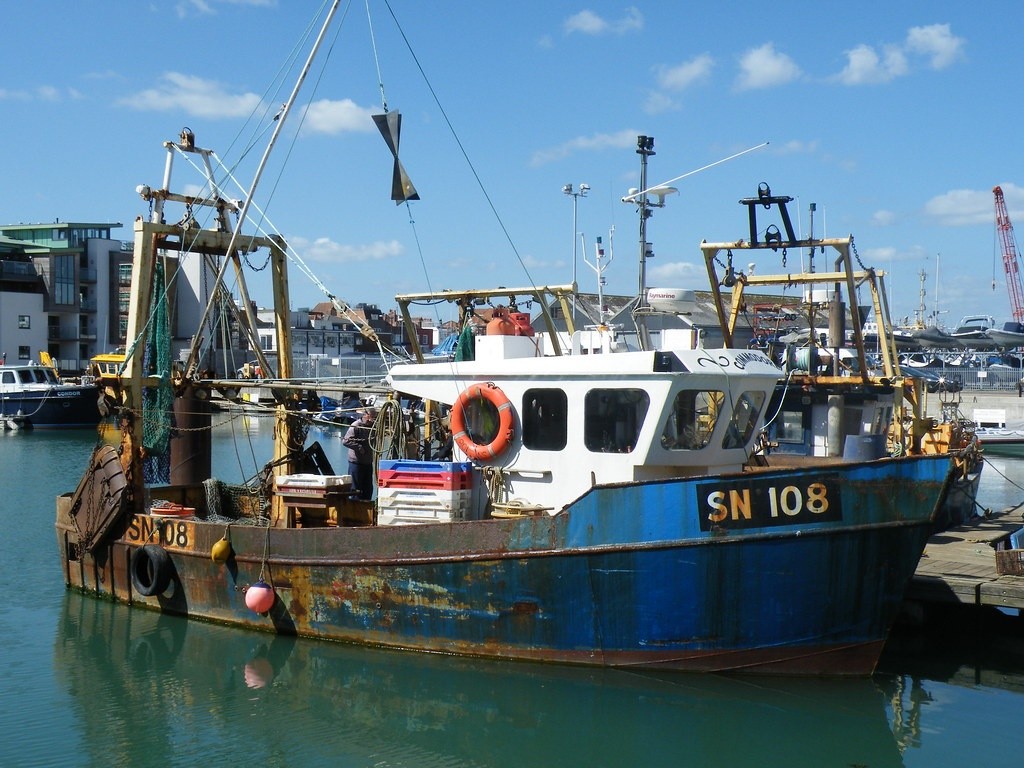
130,543 -> 172,597
449,383 -> 516,461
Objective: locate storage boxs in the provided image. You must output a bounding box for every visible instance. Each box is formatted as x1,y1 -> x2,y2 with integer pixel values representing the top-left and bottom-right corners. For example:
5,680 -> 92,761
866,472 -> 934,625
377,459 -> 472,526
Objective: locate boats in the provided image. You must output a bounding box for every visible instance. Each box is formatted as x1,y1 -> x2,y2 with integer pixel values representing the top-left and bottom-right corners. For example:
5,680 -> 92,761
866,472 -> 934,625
0,301 -> 1024,432
55,0 -> 979,679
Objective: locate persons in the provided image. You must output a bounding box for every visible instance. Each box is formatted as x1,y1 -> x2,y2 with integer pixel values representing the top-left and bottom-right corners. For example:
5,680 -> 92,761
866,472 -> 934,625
343,408 -> 387,500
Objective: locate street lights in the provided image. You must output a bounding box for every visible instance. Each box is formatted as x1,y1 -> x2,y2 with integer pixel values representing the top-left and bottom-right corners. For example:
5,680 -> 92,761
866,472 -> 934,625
561,180 -> 592,323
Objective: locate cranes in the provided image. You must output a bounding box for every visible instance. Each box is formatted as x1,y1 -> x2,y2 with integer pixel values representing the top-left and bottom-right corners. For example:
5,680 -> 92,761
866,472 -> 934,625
992,185 -> 1024,326
